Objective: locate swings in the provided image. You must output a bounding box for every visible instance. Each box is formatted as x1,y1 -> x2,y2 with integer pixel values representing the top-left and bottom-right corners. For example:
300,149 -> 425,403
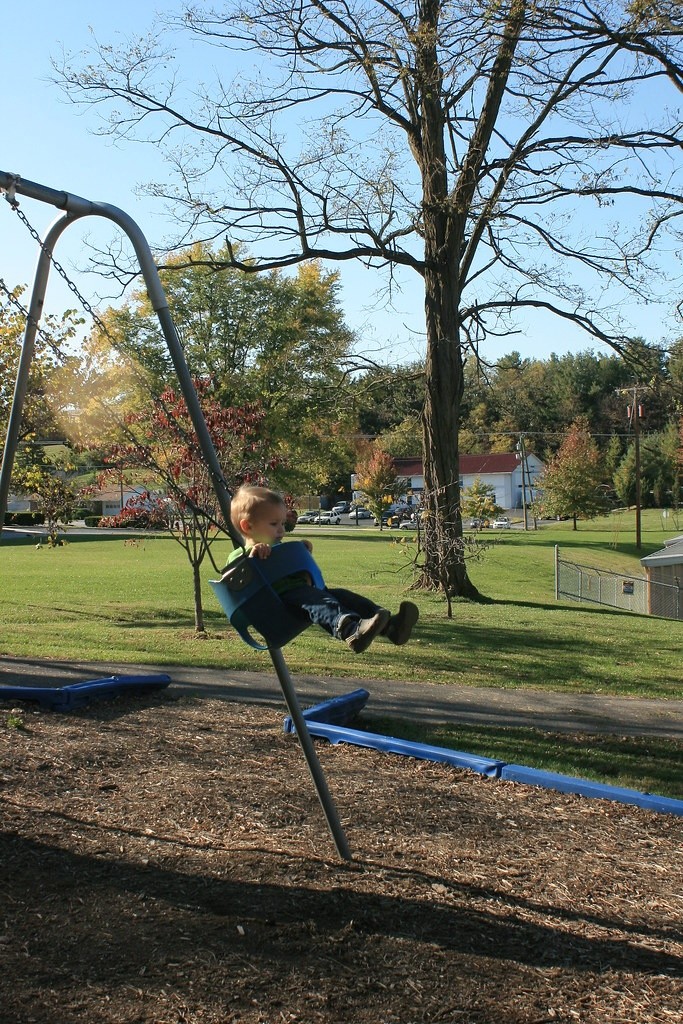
0,207 -> 326,653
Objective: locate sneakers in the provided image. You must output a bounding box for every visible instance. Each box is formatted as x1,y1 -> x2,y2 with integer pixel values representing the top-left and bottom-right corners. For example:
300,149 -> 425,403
387,601 -> 419,645
345,608 -> 389,654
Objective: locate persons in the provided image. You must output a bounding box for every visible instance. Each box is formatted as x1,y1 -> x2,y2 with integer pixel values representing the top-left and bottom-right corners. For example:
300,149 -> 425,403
221,483 -> 418,654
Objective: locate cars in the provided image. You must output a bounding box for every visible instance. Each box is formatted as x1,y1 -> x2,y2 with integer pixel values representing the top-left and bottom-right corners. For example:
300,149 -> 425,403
493,517 -> 511,530
349,508 -> 371,520
373,506 -> 425,530
297,511 -> 319,524
331,501 -> 349,513
537,508 -> 569,521
470,516 -> 490,529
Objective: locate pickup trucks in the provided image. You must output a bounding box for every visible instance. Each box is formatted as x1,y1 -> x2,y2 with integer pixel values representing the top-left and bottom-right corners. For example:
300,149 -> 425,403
314,511 -> 342,526
